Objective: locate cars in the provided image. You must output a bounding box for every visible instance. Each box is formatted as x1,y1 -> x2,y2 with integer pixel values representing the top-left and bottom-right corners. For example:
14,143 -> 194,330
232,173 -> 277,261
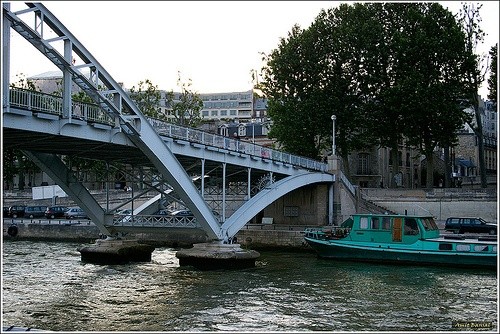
445,216 -> 497,235
3,205 -> 220,222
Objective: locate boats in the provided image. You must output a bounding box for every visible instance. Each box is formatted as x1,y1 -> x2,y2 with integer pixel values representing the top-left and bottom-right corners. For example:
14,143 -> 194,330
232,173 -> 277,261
300,213 -> 497,267
